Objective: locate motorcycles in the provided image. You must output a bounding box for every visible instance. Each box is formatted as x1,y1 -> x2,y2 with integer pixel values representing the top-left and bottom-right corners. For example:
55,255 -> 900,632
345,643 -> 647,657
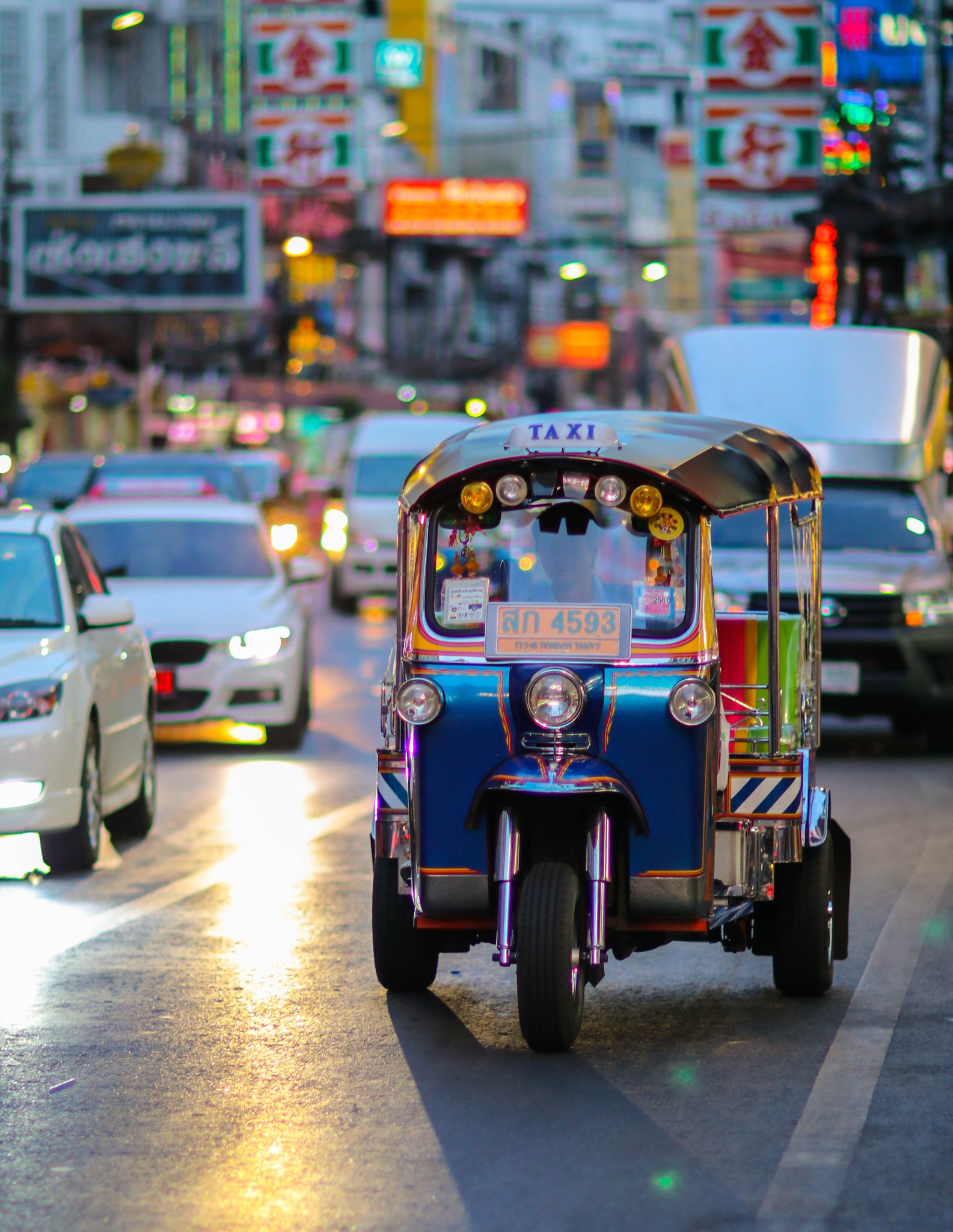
364,409 -> 854,1055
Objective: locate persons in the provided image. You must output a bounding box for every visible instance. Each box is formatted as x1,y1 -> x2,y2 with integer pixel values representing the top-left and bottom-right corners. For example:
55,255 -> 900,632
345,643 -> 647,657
519,503 -> 634,605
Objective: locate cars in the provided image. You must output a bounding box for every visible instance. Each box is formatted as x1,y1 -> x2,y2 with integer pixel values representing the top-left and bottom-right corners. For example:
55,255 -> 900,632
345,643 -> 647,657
0,450 -> 328,875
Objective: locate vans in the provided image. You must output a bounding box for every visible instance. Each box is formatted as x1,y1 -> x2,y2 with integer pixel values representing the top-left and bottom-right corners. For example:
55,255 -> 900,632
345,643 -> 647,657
647,322 -> 953,744
320,407 -> 497,613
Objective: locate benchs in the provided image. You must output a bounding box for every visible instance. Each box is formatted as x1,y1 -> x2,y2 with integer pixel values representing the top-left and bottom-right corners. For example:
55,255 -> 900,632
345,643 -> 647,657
379,611 -> 807,760
